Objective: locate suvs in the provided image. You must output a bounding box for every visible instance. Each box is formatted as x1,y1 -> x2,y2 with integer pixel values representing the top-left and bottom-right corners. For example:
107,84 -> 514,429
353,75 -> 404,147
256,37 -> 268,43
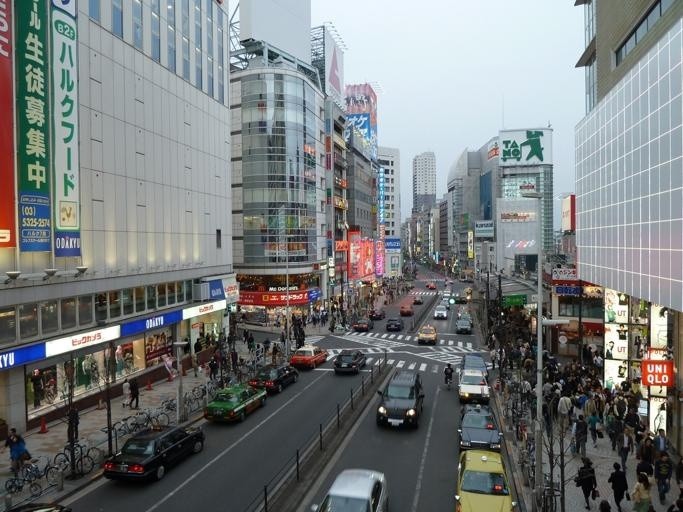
377,369 -> 425,429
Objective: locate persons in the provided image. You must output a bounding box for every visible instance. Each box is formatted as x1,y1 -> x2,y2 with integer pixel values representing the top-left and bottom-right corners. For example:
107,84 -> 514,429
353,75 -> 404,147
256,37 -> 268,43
443,362 -> 456,392
182,263 -> 415,390
30,369 -> 43,409
81,355 -> 90,389
487,303 -> 683,512
121,379 -> 130,408
9,434 -> 25,470
4,427 -> 25,470
114,345 -> 123,377
65,404 -> 78,441
128,379 -> 138,408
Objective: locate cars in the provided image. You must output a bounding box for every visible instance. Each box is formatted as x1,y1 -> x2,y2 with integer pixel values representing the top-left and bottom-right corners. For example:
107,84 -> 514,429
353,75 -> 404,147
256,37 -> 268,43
461,353 -> 490,382
457,369 -> 492,405
248,363 -> 300,393
454,449 -> 518,512
289,344 -> 329,369
310,468 -> 389,512
104,424 -> 205,482
353,270 -> 472,345
2,503 -> 72,512
457,404 -> 504,453
203,384 -> 268,422
334,349 -> 367,374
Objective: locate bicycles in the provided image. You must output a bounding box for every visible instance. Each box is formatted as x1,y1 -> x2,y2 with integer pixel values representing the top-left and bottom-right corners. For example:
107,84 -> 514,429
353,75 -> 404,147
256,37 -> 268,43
64,437 -> 102,465
502,376 -> 561,512
161,392 -> 192,415
54,444 -> 94,475
444,378 -> 453,390
5,464 -> 42,497
169,389 -> 200,412
113,410 -> 150,437
192,367 -> 252,399
125,407 -> 159,430
22,453 -> 60,486
140,406 -> 170,425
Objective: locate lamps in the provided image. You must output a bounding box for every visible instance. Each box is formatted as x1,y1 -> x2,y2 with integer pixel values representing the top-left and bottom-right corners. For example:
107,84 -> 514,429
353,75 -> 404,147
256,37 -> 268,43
73,265 -> 89,279
41,269 -> 59,282
3,270 -> 22,286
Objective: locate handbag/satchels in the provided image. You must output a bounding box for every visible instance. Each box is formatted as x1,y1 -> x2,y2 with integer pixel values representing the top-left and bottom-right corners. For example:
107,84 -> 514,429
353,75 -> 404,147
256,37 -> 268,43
592,488 -> 599,500
24,449 -> 31,460
626,493 -> 631,501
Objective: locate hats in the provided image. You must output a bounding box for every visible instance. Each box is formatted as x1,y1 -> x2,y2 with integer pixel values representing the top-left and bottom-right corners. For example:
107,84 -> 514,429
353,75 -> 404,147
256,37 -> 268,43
581,457 -> 593,464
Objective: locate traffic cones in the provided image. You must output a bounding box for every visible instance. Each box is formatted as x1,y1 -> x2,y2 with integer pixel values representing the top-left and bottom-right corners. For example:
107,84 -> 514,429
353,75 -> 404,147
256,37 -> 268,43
37,417 -> 49,434
494,377 -> 501,391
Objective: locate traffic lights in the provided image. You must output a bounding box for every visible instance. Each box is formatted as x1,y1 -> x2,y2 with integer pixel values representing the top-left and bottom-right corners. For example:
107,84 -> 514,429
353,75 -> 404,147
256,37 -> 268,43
476,264 -> 479,273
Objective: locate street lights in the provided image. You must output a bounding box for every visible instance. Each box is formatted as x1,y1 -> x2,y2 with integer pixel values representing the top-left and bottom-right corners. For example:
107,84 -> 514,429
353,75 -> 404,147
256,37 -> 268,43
521,192 -> 570,512
172,341 -> 188,425
285,235 -> 295,356
